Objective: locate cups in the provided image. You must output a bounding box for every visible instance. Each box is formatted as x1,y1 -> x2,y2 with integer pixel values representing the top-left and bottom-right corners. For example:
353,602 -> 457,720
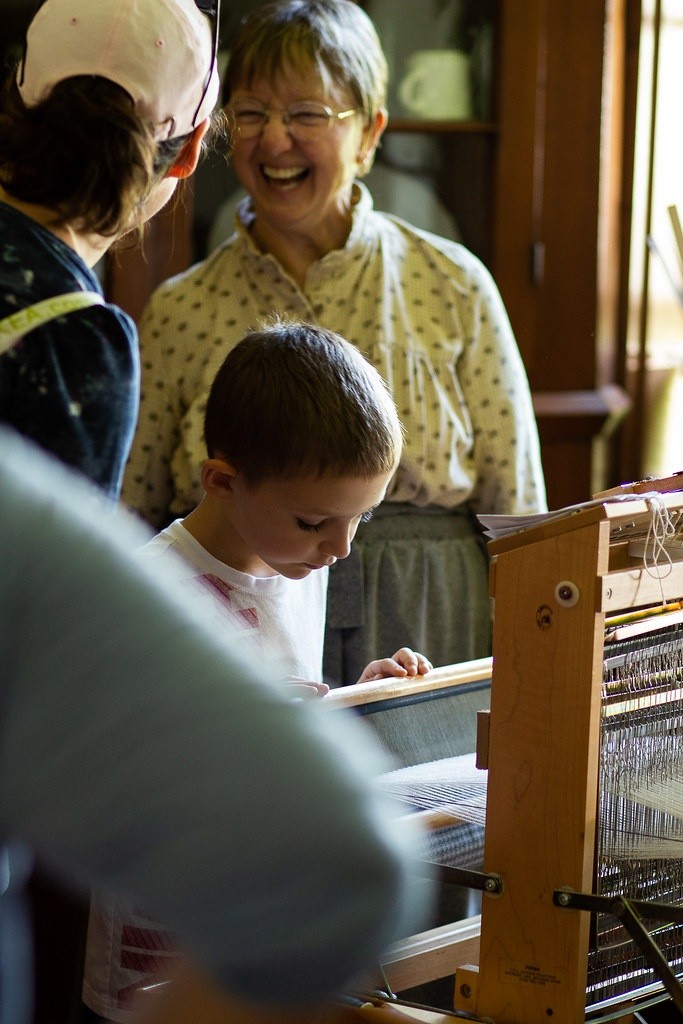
399,49 -> 469,119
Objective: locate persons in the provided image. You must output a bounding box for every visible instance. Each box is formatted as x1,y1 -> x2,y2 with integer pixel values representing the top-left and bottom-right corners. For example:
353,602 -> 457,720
0,0 -> 224,1024
120,0 -> 551,688
78,320 -> 435,1023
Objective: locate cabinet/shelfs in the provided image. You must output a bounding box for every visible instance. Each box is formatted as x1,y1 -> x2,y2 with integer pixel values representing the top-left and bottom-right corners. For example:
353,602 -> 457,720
111,1 -> 503,323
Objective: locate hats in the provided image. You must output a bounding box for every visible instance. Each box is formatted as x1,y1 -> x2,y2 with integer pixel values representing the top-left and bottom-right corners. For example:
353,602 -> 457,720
13,0 -> 225,138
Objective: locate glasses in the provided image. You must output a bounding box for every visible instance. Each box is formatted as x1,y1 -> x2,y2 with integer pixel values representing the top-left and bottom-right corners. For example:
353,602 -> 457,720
191,0 -> 221,127
223,96 -> 361,141
